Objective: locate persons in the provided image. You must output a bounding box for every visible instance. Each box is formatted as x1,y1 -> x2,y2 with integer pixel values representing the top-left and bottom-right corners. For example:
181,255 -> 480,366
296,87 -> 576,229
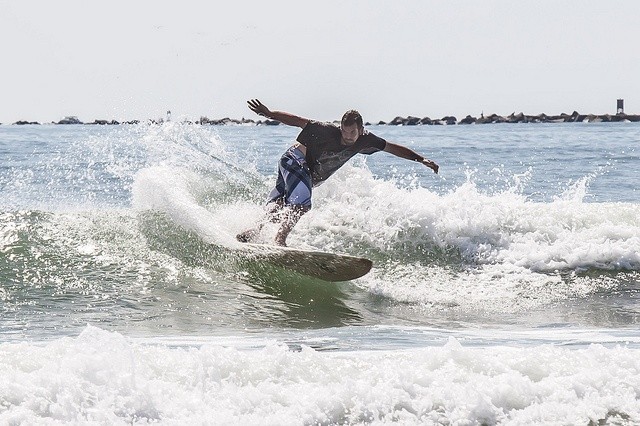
235,98 -> 441,248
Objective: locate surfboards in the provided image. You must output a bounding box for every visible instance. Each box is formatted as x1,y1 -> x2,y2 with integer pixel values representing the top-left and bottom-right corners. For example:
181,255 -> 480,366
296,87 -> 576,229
210,241 -> 372,282
